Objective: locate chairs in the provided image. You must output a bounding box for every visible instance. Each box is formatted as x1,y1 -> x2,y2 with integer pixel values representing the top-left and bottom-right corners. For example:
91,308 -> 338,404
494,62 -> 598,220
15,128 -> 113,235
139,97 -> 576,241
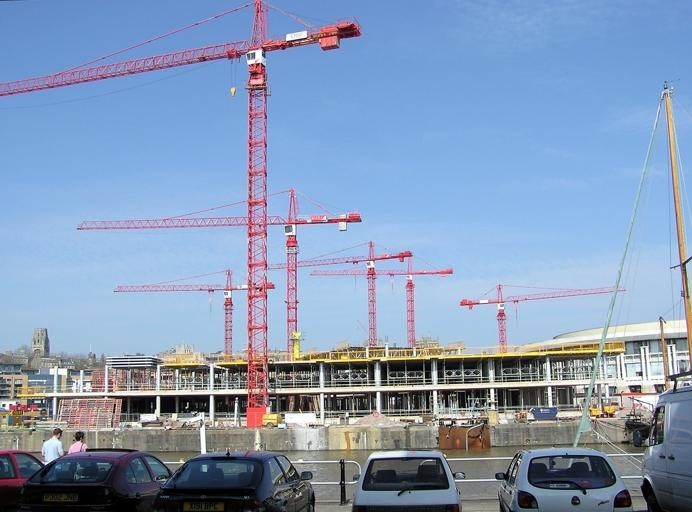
207,468 -> 254,485
375,465 -> 440,483
529,461 -> 589,477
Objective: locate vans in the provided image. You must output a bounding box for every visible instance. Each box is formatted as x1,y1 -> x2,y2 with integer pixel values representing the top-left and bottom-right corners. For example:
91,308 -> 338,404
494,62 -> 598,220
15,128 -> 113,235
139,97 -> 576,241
631,381 -> 692,512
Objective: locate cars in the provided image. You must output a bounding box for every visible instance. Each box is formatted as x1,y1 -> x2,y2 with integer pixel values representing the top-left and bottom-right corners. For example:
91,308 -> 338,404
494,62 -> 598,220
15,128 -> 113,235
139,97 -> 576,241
350,449 -> 467,511
494,447 -> 635,512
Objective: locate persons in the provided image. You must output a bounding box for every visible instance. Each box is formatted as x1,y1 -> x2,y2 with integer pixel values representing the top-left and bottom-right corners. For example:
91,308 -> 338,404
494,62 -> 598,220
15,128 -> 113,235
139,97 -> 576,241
41,428 -> 64,465
68,431 -> 88,454
